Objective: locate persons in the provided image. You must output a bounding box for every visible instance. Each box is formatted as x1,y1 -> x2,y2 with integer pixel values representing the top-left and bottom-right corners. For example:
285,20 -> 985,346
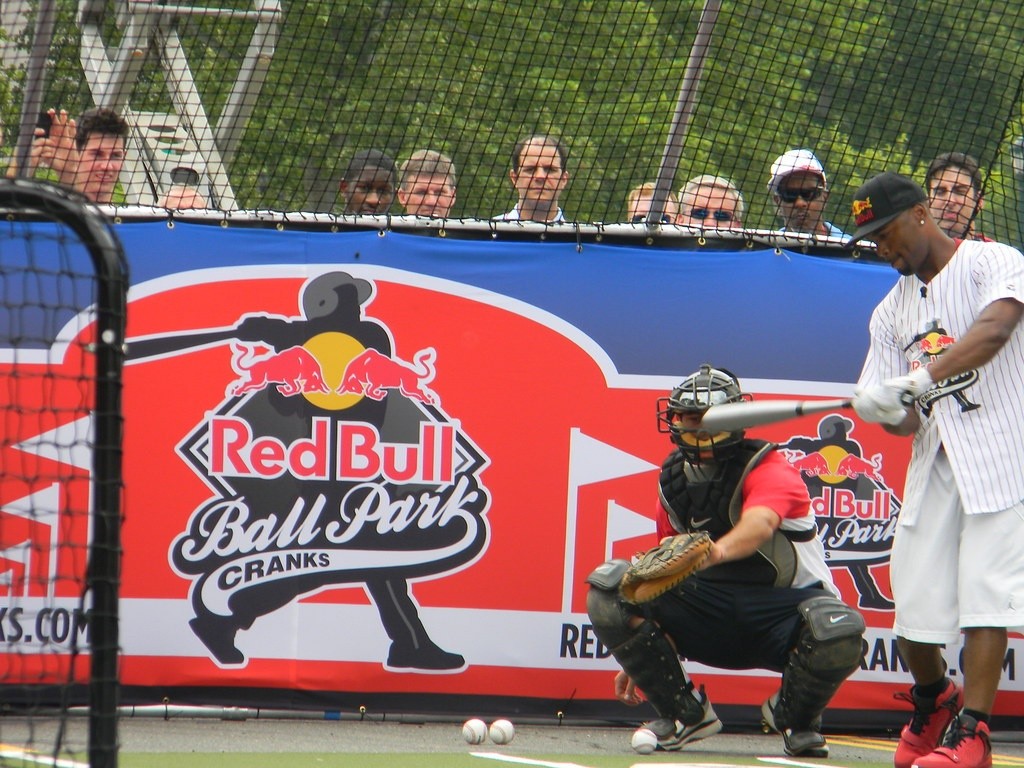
158,188 -> 205,207
396,147 -> 460,217
681,175 -> 747,231
924,155 -> 1004,242
339,154 -> 398,214
77,109 -> 130,202
849,173 -> 1024,768
493,133 -> 570,222
770,145 -> 841,237
4,109 -> 81,188
626,180 -> 679,221
587,367 -> 864,758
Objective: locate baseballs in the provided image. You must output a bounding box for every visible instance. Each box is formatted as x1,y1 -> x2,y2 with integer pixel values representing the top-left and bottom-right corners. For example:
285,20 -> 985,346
462,718 -> 488,745
631,728 -> 657,754
489,719 -> 516,744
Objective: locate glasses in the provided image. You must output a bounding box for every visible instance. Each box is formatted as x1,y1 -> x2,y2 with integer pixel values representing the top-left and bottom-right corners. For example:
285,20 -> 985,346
777,187 -> 823,203
632,215 -> 671,224
683,209 -> 734,221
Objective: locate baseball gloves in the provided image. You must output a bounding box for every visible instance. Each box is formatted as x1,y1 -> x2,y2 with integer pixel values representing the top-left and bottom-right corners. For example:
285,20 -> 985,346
619,533 -> 718,607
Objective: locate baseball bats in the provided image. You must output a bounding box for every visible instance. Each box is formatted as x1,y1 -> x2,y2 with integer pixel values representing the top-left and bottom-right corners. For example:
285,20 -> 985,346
702,386 -> 915,432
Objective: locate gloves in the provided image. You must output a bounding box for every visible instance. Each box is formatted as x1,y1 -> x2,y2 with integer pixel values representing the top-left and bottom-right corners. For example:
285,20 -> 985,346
850,366 -> 934,425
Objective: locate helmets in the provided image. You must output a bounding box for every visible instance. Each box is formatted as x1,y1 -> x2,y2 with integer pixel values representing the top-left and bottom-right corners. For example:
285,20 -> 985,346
666,369 -> 743,465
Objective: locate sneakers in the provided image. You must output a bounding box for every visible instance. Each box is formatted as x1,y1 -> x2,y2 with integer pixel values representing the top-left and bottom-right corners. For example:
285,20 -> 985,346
761,687 -> 831,756
911,704 -> 992,768
654,684 -> 723,750
894,678 -> 963,768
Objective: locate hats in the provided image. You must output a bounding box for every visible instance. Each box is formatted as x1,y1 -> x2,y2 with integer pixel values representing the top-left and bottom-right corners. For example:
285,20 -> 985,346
766,149 -> 827,190
843,172 -> 925,247
